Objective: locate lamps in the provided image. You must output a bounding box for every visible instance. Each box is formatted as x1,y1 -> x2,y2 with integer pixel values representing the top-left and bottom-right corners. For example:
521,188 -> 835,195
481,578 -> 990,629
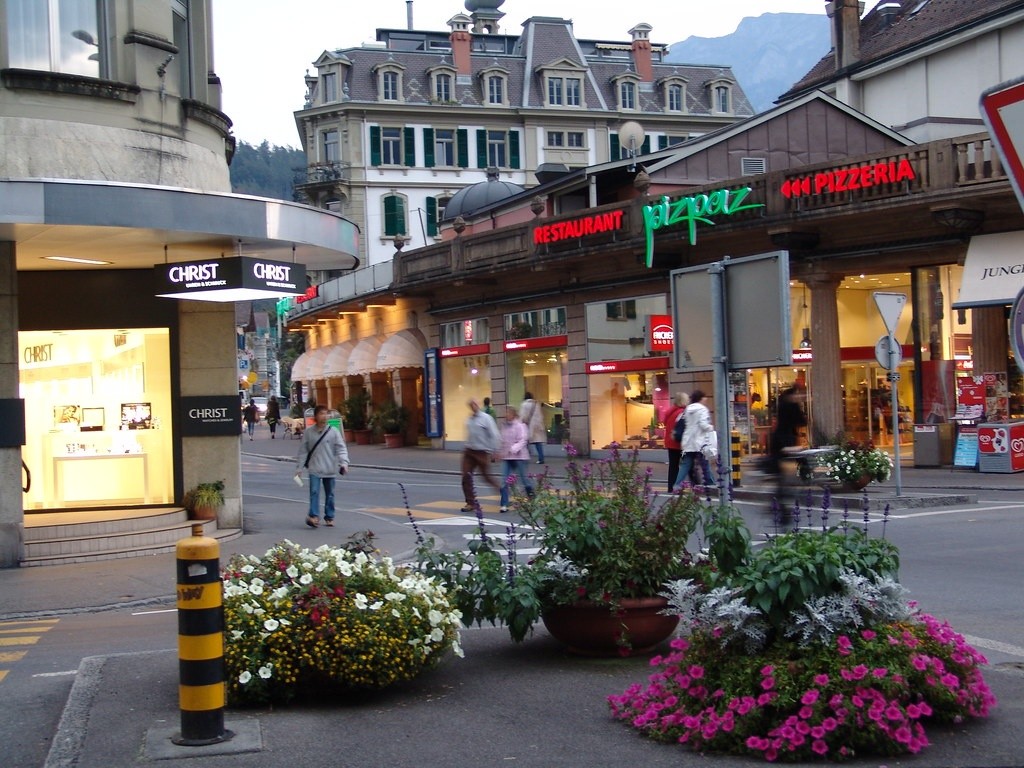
799,308 -> 812,348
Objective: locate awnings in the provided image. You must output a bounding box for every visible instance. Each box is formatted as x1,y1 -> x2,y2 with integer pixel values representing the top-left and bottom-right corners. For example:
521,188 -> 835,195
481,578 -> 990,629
951,229 -> 1024,311
322,340 -> 356,378
346,333 -> 394,374
305,345 -> 332,379
376,328 -> 428,370
291,348 -> 312,381
278,396 -> 286,398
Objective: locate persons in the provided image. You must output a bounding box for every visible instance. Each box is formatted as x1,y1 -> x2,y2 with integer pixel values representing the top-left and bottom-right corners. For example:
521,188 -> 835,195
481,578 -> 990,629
461,400 -> 503,511
483,397 -> 495,417
519,391 -> 547,464
243,399 -> 257,440
266,395 -> 281,439
295,405 -> 350,528
762,386 -> 808,452
661,390 -> 715,494
497,403 -> 538,511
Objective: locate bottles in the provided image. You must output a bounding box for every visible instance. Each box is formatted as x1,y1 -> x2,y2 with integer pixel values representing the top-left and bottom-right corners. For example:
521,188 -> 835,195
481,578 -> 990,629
925,397 -> 945,424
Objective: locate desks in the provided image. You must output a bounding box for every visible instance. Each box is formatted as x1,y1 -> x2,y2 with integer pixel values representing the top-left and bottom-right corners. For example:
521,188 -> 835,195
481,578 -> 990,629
753,426 -> 772,455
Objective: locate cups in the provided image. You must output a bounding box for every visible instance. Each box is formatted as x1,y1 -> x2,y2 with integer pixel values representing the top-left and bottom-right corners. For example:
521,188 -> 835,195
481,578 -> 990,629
293,474 -> 304,488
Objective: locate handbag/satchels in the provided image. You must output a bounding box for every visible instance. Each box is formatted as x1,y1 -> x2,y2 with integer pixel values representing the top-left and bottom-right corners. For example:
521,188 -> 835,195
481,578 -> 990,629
254,411 -> 260,424
698,430 -> 717,459
673,413 -> 685,442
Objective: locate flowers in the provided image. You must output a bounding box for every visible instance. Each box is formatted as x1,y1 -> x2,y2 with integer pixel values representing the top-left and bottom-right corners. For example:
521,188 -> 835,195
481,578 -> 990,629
220,441 -> 997,762
796,430 -> 894,484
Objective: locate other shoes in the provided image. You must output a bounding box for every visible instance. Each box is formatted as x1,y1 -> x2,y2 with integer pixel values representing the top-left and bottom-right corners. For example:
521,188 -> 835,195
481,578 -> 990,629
461,504 -> 475,512
325,519 -> 333,527
272,435 -> 275,439
536,460 -> 544,465
306,516 -> 320,528
500,504 -> 508,512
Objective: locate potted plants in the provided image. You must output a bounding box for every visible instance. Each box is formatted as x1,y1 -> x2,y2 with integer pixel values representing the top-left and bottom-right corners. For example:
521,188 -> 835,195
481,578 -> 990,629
337,388 -> 410,448
182,480 -> 225,520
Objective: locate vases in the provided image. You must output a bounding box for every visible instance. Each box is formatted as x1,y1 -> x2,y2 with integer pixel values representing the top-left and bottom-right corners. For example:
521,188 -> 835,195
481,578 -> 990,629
540,596 -> 681,659
814,471 -> 872,493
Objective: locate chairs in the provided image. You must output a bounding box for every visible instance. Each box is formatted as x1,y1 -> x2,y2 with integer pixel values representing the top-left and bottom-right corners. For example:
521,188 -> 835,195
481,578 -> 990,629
277,416 -> 304,440
